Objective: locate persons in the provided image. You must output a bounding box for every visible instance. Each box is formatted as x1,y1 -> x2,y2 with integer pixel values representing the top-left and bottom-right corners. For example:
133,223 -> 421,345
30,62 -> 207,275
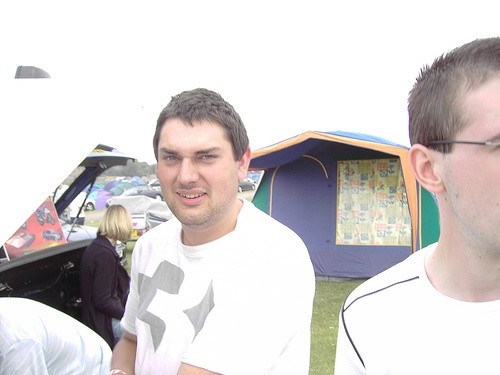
107,89 -> 316,375
333,37 -> 500,375
0,205 -> 132,375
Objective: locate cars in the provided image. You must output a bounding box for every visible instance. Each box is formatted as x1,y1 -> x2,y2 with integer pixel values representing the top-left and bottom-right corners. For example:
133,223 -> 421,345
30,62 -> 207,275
0,144 -> 257,323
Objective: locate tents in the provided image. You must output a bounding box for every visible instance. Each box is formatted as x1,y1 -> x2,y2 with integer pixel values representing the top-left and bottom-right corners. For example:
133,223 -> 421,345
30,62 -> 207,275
247,131 -> 441,282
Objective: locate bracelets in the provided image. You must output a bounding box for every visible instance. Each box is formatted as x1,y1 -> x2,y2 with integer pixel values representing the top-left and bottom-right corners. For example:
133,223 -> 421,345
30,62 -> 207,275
107,369 -> 127,375
